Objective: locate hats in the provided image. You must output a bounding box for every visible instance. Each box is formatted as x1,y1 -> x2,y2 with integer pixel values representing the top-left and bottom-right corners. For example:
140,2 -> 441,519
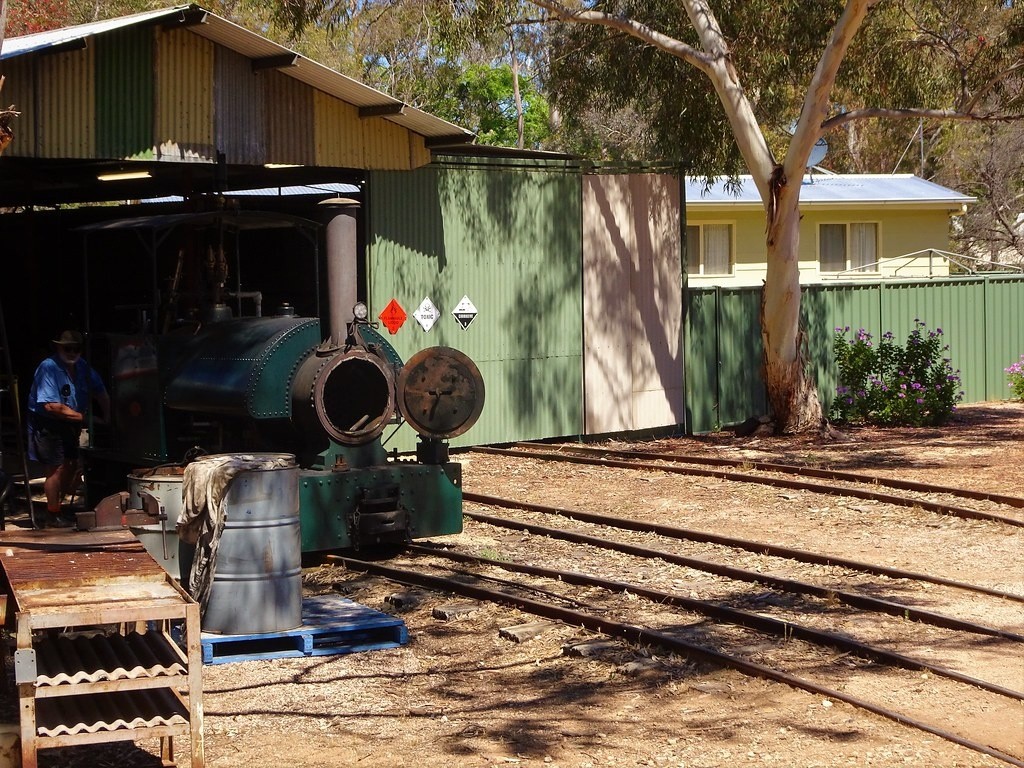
51,330 -> 84,345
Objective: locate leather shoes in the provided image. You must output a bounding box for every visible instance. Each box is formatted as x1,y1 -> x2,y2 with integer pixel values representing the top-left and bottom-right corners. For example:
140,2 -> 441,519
47,515 -> 75,528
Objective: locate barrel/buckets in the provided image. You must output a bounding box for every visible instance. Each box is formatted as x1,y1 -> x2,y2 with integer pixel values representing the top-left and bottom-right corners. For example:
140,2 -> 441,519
126,472 -> 183,584
194,452 -> 303,635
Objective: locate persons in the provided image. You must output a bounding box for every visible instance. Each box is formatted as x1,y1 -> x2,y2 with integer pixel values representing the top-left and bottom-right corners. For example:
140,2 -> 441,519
27,330 -> 110,517
